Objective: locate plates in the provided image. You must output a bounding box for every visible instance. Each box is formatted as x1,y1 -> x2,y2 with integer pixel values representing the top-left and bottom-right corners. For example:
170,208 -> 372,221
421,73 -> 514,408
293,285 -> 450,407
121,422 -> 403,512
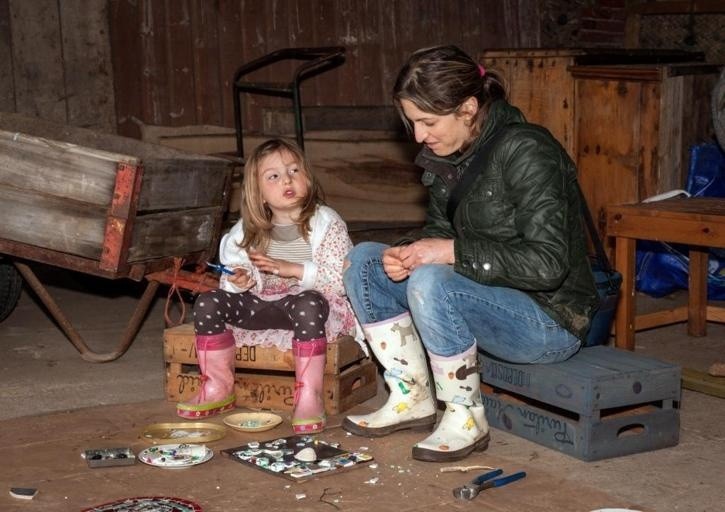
140,443 -> 214,470
224,414 -> 280,434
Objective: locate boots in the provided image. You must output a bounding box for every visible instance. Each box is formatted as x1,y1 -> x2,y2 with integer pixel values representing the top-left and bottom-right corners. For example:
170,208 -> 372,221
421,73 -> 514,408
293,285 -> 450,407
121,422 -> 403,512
342,311 -> 438,439
175,328 -> 238,421
411,337 -> 491,463
291,336 -> 328,436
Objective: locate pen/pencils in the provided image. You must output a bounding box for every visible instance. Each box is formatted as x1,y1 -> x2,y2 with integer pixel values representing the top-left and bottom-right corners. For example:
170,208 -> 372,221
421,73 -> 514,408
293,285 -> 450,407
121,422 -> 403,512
206,263 -> 236,275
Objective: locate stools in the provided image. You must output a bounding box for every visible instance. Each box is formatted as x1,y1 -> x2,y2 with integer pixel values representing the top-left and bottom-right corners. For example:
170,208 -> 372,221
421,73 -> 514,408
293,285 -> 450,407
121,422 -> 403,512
605,194 -> 725,352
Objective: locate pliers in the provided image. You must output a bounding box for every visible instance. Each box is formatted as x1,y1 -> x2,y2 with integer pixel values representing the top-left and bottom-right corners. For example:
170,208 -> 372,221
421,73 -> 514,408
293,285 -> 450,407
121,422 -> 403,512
452,468 -> 526,500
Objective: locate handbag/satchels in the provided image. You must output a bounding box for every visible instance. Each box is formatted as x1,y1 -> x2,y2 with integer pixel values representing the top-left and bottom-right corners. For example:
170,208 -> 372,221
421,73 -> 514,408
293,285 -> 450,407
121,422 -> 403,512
581,269 -> 623,348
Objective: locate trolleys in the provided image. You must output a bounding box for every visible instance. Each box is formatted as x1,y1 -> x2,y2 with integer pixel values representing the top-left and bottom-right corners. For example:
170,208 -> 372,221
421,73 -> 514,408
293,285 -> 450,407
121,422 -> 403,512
204,43 -> 348,184
0,114 -> 235,364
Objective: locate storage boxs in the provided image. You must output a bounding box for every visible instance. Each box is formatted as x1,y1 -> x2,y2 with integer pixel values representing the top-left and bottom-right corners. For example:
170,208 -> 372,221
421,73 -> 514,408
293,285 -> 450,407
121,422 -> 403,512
163,318 -> 377,413
477,343 -> 682,462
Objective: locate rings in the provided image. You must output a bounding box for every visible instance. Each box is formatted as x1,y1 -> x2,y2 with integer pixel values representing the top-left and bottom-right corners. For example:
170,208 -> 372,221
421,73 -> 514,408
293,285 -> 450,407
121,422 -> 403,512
272,267 -> 279,274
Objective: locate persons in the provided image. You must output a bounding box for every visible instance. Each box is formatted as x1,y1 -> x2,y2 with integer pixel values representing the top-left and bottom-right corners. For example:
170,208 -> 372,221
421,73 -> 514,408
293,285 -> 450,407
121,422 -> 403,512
342,45 -> 601,463
176,138 -> 355,434
